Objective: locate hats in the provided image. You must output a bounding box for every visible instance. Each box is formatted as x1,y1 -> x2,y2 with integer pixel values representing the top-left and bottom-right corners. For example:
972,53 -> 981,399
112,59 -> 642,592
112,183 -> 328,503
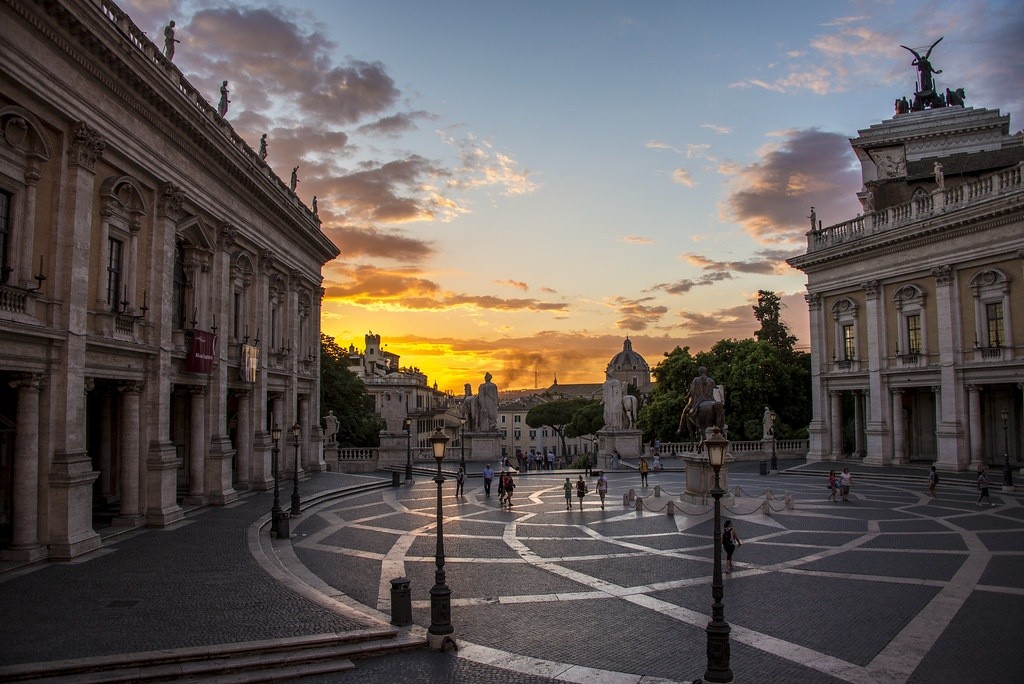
487,464 -> 491,467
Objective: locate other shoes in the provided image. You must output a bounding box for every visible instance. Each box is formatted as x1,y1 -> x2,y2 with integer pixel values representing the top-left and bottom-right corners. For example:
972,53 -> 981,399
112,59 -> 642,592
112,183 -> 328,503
843,498 -> 848,502
828,497 -> 838,502
566,507 -> 569,510
724,565 -> 735,573
498,499 -> 504,504
646,484 -> 649,486
508,504 -> 514,506
569,503 -> 572,507
990,504 -> 996,508
504,499 -> 506,504
642,484 -> 644,486
601,506 -> 604,511
976,503 -> 983,506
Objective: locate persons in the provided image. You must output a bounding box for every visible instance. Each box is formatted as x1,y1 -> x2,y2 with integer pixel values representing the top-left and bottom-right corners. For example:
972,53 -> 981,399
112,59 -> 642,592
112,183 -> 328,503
164,21 -> 181,61
763,407 -> 775,436
678,365 -> 715,434
807,209 -> 816,231
930,162 -> 943,189
312,196 -> 318,216
585,451 -> 593,477
292,165 -> 300,195
218,80 -> 231,118
260,134 -> 268,160
637,438 -> 661,487
383,387 -> 407,410
911,57 -> 936,90
454,464 -> 515,507
864,190 -> 874,212
611,447 -> 621,465
975,470 -> 995,508
602,369 -> 623,430
722,520 -> 741,574
502,449 -> 555,473
478,372 -> 499,431
326,411 -> 340,442
826,467 -> 853,503
928,466 -> 938,499
564,470 -> 607,511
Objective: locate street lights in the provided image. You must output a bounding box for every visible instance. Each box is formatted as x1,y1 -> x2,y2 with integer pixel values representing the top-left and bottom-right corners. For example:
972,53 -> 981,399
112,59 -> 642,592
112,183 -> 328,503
459,414 -> 466,474
593,436 -> 597,466
290,422 -> 301,515
270,424 -> 281,533
702,426 -> 733,683
1001,407 -> 1013,486
771,410 -> 777,470
428,427 -> 454,635
405,416 -> 413,479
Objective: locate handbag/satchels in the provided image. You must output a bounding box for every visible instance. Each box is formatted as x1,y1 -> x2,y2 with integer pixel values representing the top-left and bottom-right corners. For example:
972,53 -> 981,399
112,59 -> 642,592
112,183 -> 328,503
834,479 -> 840,488
839,487 -> 844,496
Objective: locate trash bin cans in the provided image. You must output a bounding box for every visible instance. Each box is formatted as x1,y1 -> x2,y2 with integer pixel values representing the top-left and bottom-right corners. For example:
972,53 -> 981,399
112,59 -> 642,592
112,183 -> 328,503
327,464 -> 331,472
388,577 -> 412,627
759,461 -> 767,475
393,471 -> 400,486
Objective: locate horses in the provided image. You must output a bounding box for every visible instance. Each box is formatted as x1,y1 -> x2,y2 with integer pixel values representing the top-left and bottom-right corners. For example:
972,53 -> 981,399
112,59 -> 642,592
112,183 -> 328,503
464,383 -> 479,432
946,87 -> 966,108
895,97 -> 908,114
686,384 -> 725,454
620,381 -> 639,430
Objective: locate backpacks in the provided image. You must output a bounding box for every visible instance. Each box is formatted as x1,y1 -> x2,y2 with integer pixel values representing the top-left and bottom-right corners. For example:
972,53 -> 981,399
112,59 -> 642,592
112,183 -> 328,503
722,528 -> 733,546
934,471 -> 938,483
